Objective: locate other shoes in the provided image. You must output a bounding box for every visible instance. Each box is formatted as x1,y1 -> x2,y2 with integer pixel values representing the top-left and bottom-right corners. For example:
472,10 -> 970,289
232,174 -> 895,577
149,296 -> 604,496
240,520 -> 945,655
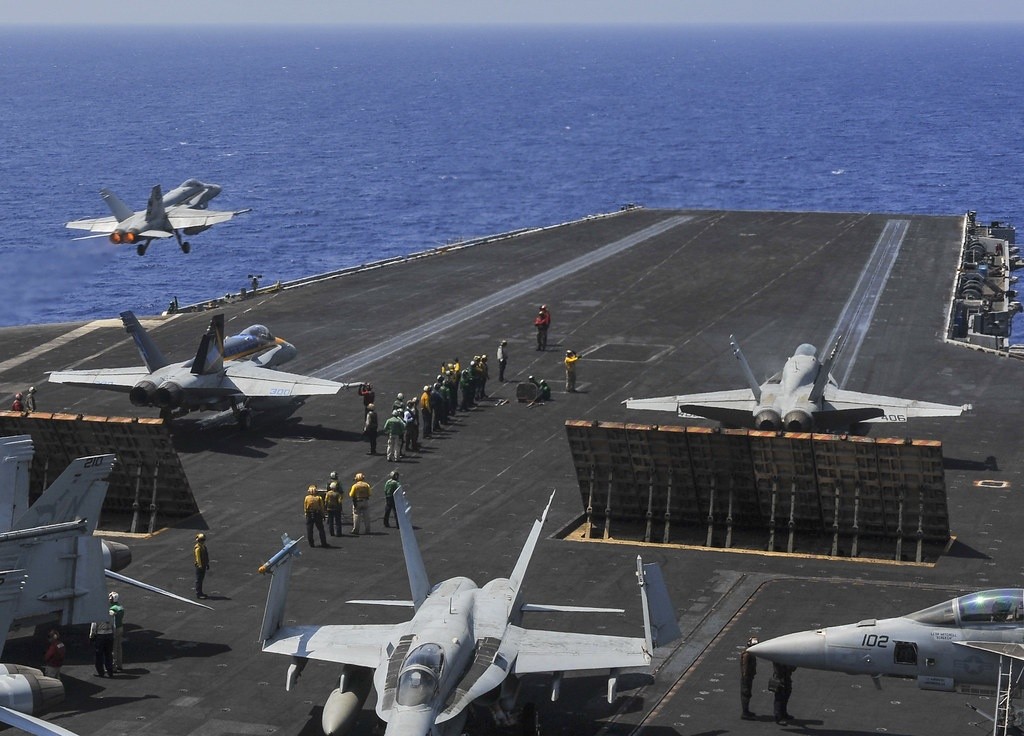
93,673 -> 113,677
387,458 -> 401,462
400,454 -> 404,458
104,668 -> 121,672
197,593 -> 208,598
366,450 -> 376,455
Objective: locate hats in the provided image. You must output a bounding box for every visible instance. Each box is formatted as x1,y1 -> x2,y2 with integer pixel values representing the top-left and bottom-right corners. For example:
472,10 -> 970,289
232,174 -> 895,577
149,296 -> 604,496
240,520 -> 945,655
529,375 -> 535,380
540,380 -> 545,385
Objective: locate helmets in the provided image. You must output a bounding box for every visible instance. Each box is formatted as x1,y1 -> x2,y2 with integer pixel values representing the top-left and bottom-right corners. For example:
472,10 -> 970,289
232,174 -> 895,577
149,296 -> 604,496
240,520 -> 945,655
423,363 -> 455,392
540,305 -> 547,310
29,387 -> 37,394
15,393 -> 22,401
500,340 -> 506,346
367,403 -> 375,410
470,354 -> 488,368
392,393 -> 418,416
195,533 -> 206,542
390,471 -> 399,479
538,311 -> 544,316
330,471 -> 338,480
355,472 -> 364,481
109,591 -> 119,603
330,482 -> 338,490
364,382 -> 372,391
308,485 -> 318,496
566,350 -> 572,355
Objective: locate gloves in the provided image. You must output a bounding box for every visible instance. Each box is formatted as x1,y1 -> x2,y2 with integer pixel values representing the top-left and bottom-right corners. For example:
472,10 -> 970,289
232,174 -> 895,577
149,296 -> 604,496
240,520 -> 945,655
578,354 -> 582,358
573,351 -> 576,354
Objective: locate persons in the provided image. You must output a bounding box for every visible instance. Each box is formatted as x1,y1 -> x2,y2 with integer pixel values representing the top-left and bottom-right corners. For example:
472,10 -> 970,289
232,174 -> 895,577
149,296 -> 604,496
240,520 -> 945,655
773,662 -> 797,725
535,305 -> 551,351
740,637 -> 757,720
304,471 -> 345,548
25,387 -> 38,412
383,471 -> 400,529
564,349 -> 583,392
12,393 -> 24,412
359,355 -> 488,461
496,340 -> 508,382
43,631 -> 66,679
89,592 -> 124,676
193,534 -> 210,600
348,474 -> 371,534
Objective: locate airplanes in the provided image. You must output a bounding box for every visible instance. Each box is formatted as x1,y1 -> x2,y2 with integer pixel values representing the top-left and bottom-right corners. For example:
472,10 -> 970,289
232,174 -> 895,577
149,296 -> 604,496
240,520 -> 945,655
43,308 -> 368,435
0,662 -> 79,736
253,481 -> 657,736
61,178 -> 255,257
745,587 -> 1024,701
0,433 -> 215,661
619,331 -> 975,436
